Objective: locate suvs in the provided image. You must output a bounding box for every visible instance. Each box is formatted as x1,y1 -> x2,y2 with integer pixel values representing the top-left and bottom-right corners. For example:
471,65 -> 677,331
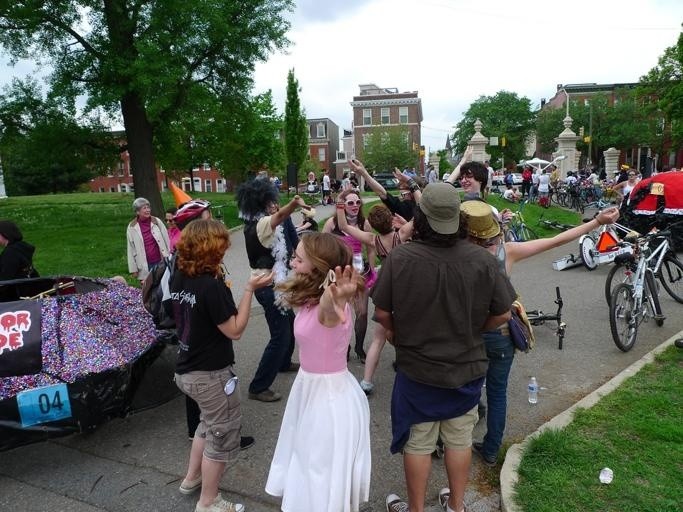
369,170 -> 401,191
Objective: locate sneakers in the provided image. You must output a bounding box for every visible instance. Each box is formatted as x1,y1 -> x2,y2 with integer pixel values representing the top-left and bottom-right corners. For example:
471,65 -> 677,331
179,474 -> 202,495
360,381 -> 373,399
194,493 -> 245,512
287,363 -> 300,372
386,493 -> 410,512
249,390 -> 281,402
472,442 -> 497,467
438,488 -> 467,512
239,437 -> 255,450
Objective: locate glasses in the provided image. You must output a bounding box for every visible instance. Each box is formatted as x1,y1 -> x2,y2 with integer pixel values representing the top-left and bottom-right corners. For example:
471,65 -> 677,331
344,199 -> 361,207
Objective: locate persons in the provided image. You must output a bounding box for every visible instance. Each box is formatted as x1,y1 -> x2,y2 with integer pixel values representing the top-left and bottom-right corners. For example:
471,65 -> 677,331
127,145 -> 682,512
0,218 -> 36,281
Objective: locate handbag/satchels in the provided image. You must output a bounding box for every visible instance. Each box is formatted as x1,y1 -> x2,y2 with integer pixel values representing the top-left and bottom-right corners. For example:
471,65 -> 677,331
507,314 -> 531,352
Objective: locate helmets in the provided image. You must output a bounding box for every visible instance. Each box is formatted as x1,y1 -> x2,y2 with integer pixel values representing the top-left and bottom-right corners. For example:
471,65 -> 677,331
172,199 -> 211,231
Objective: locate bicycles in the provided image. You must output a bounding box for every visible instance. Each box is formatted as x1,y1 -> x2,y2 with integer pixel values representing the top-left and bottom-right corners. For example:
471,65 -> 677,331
506,180 -> 683,350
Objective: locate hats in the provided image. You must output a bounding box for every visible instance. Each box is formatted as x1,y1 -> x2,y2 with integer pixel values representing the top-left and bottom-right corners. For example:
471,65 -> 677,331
419,183 -> 460,234
459,199 -> 500,240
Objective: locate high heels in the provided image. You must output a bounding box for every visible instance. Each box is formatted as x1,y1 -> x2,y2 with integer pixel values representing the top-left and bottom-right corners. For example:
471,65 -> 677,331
353,347 -> 366,364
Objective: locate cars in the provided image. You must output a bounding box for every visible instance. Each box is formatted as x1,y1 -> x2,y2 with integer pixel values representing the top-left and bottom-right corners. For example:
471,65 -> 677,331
494,173 -> 526,186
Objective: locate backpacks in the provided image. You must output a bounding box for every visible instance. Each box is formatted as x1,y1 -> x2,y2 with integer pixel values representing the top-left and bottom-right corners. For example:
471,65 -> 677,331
141,252 -> 184,330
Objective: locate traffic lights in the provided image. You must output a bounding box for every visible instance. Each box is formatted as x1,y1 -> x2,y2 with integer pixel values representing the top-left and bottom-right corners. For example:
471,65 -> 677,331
579,125 -> 584,138
